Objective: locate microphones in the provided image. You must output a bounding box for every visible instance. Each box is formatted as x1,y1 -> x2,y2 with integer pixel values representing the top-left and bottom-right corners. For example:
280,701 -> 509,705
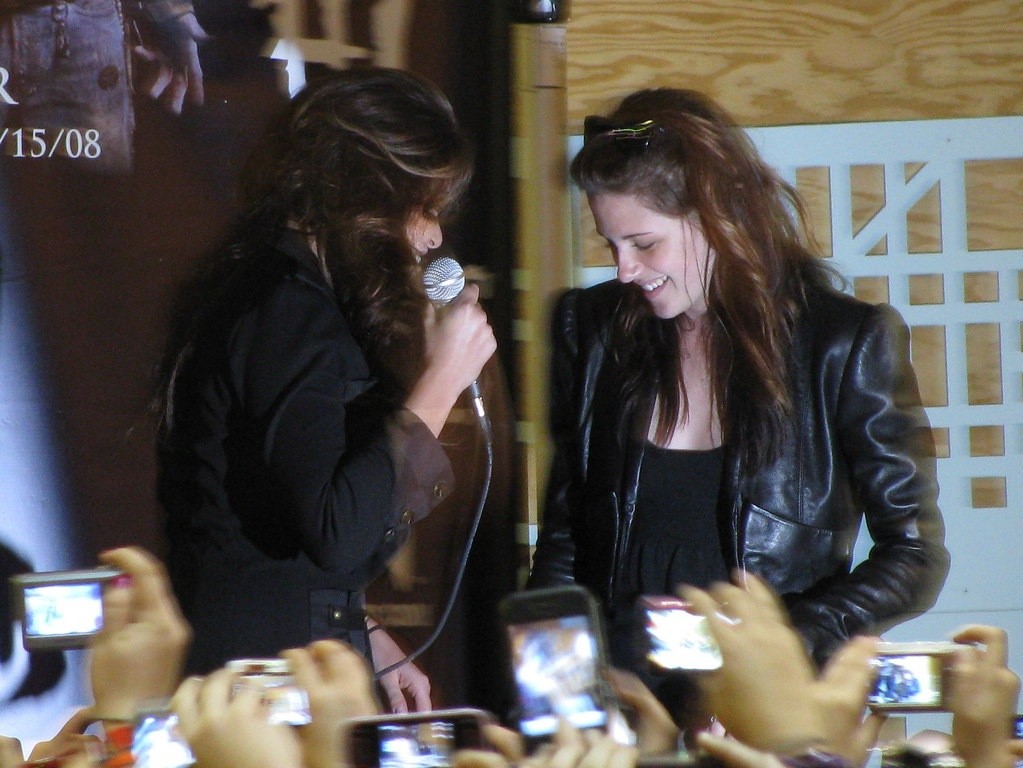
421,256 -> 493,447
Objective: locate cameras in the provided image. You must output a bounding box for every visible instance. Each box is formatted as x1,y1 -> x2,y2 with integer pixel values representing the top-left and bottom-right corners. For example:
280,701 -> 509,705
642,595 -> 740,672
868,640 -> 978,713
15,567 -> 131,650
130,710 -> 197,768
222,657 -> 313,726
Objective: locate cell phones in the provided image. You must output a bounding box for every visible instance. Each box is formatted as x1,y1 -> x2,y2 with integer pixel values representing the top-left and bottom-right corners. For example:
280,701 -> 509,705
500,586 -> 614,756
344,709 -> 492,768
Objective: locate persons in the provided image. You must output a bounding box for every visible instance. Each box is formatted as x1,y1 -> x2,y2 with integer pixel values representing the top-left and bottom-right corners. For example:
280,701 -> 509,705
152,68 -> 498,714
524,84 -> 951,726
0,548 -> 1023,768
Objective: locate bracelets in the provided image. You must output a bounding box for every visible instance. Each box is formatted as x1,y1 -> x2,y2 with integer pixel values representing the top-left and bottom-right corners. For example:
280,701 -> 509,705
366,623 -> 386,633
